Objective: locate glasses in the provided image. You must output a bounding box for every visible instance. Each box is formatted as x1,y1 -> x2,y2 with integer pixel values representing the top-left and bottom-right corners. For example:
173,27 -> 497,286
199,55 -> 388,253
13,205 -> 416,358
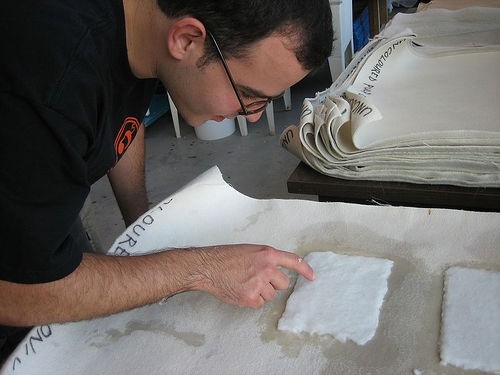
206,31 -> 283,116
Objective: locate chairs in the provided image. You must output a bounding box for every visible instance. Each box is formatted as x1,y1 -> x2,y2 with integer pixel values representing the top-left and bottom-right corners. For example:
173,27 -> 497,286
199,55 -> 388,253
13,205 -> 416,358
265,0 -> 355,136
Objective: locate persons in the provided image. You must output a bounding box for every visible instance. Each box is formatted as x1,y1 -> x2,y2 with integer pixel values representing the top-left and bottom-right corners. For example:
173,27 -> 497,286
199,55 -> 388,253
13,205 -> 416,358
0,0 -> 336,369
